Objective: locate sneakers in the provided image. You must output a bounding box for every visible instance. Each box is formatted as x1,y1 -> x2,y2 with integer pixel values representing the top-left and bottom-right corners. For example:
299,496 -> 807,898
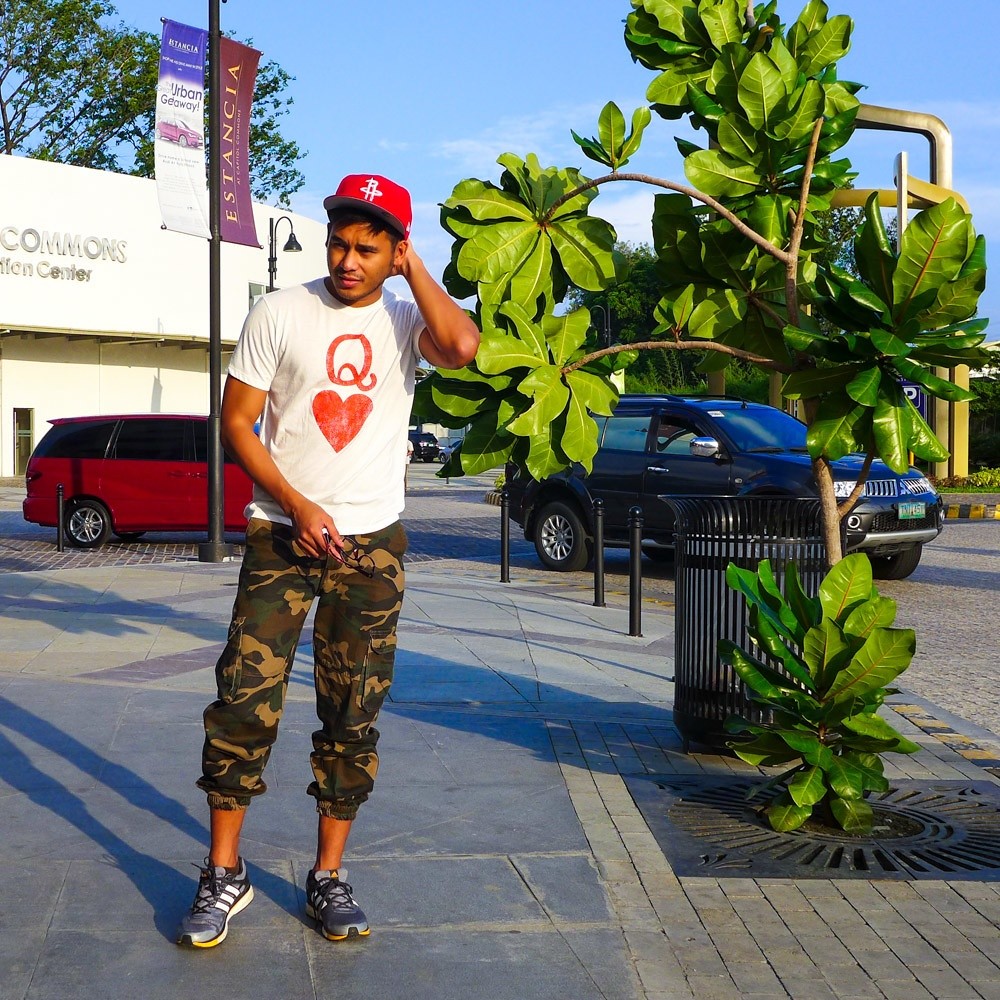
176,855 -> 254,949
304,867 -> 370,941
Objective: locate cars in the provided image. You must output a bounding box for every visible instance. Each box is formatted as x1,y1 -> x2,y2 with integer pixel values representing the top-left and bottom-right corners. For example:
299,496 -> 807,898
438,439 -> 464,464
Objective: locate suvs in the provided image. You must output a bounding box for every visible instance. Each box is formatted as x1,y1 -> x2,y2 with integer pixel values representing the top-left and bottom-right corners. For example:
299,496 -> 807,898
409,433 -> 440,463
503,392 -> 947,580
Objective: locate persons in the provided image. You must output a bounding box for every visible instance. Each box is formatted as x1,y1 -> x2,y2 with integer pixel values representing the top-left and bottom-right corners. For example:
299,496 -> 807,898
403,440 -> 414,493
177,175 -> 479,948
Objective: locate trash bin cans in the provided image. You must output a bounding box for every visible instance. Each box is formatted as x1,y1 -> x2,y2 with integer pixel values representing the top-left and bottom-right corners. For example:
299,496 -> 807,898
658,495 -> 876,754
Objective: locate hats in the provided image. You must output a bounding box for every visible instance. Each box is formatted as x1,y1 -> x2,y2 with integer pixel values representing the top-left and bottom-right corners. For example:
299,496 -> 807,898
323,173 -> 413,247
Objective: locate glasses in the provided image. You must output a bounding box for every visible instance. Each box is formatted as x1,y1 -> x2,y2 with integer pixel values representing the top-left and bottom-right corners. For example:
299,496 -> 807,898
325,532 -> 376,579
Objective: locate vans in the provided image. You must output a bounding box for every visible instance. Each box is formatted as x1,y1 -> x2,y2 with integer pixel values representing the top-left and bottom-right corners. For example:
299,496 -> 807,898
22,414 -> 261,549
